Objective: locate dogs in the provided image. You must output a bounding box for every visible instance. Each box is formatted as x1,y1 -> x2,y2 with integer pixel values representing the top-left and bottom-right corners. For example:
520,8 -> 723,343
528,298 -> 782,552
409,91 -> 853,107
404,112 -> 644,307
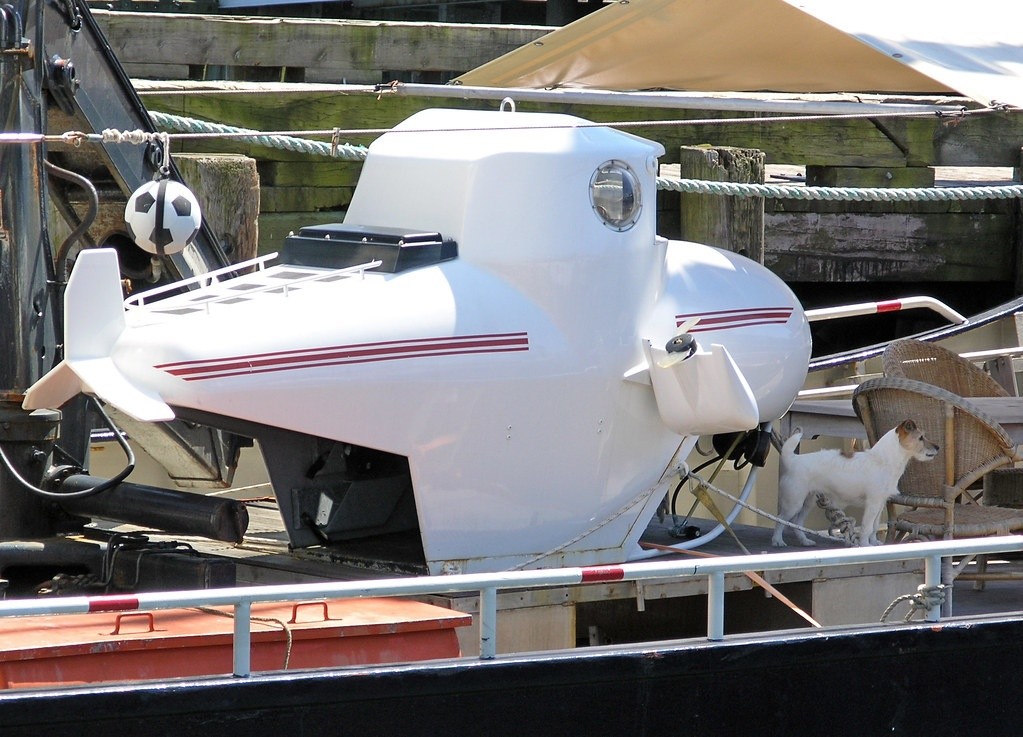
771,417 -> 940,548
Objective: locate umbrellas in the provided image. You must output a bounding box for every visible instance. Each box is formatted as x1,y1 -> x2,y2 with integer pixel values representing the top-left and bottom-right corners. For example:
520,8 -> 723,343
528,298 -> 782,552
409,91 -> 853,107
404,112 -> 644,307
449,1 -> 985,118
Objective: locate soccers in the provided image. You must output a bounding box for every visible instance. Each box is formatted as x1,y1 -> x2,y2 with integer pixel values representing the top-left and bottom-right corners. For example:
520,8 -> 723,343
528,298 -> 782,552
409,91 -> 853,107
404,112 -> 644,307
124,183 -> 202,253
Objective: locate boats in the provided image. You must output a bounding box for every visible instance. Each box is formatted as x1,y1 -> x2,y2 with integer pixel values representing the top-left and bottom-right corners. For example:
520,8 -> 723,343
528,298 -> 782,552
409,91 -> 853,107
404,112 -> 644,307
32,0 -> 1023,300
2,0 -> 1023,724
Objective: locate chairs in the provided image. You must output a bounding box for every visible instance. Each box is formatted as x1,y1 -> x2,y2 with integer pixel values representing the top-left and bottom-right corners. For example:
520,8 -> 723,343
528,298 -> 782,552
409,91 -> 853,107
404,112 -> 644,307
851,378 -> 1023,618
881,339 -> 1023,594
